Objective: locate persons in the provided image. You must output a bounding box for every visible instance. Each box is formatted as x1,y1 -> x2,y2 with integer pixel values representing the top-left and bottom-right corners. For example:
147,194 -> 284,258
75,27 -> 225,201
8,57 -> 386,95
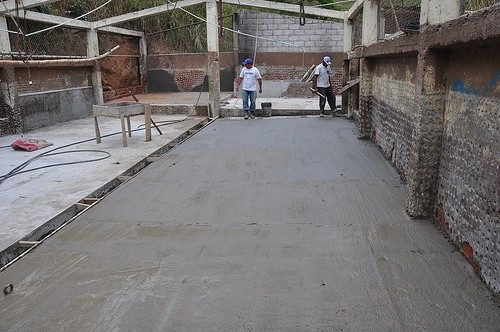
236,58 -> 262,119
312,56 -> 340,117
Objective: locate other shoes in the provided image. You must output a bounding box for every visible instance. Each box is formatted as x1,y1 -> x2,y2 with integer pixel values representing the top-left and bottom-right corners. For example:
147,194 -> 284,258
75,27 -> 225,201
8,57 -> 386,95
244,115 -> 249,119
331,112 -> 340,117
250,114 -> 255,119
320,113 -> 324,117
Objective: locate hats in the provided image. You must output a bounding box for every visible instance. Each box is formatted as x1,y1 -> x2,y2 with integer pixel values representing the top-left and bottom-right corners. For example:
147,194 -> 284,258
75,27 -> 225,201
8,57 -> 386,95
323,56 -> 331,65
242,58 -> 252,64
